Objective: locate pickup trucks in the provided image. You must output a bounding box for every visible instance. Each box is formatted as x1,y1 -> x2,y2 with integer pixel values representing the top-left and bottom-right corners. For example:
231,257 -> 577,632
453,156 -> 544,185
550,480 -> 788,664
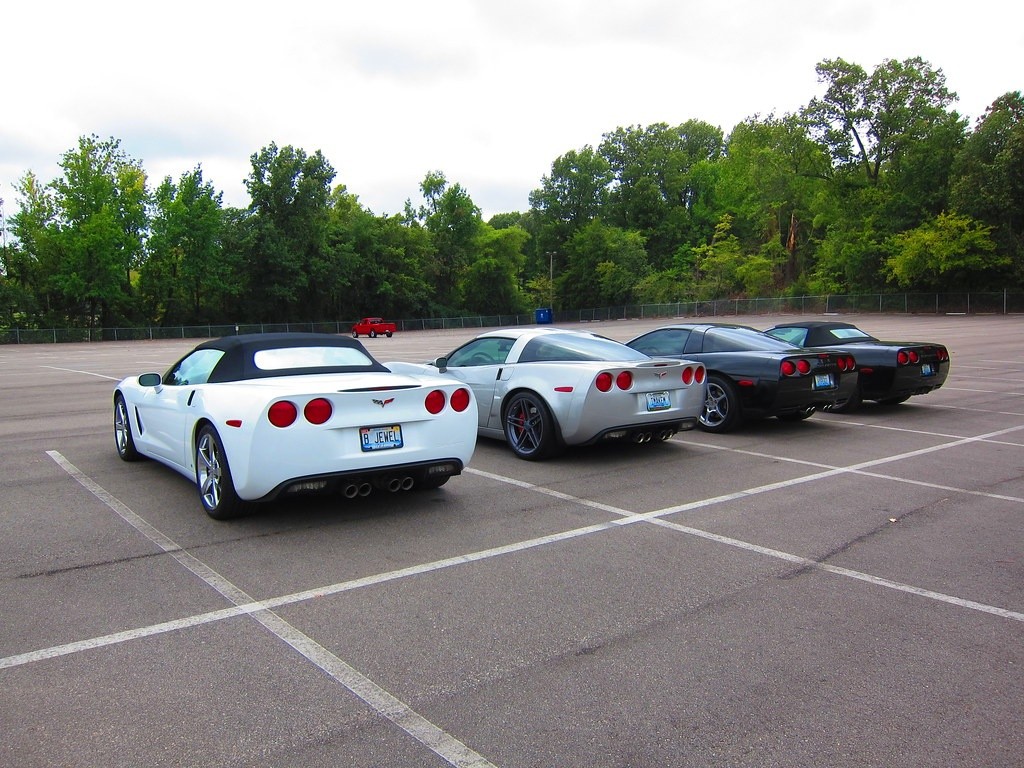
351,318 -> 396,338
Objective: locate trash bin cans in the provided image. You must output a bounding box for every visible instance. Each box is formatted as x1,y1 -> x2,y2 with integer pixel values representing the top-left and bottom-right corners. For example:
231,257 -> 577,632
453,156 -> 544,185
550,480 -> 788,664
536,308 -> 552,324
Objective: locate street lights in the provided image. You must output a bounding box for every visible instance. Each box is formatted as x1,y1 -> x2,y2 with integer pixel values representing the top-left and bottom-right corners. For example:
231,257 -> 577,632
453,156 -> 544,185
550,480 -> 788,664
546,250 -> 558,309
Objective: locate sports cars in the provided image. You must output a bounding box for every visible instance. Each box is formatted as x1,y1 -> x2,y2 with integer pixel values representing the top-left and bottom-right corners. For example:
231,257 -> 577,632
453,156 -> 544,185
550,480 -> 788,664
628,322 -> 860,434
424,328 -> 708,461
112,331 -> 478,522
763,322 -> 950,412
